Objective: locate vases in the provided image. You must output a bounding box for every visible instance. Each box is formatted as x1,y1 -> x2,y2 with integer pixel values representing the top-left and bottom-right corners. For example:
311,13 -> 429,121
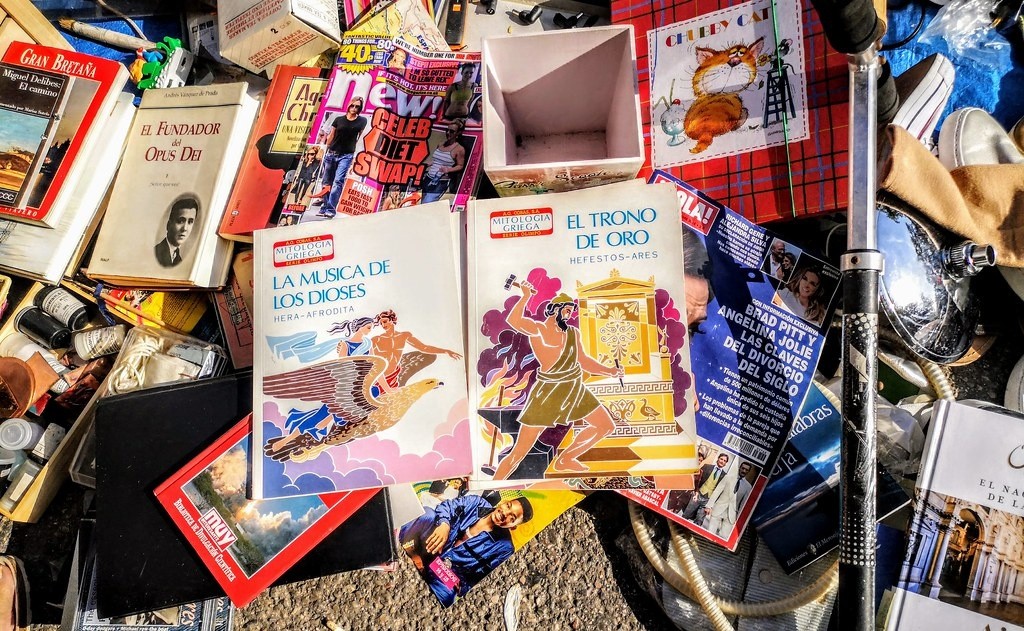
482,25 -> 645,198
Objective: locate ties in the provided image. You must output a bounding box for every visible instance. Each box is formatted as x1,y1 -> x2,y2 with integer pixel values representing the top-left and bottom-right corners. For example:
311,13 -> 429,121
734,478 -> 742,494
174,248 -> 180,266
714,467 -> 720,479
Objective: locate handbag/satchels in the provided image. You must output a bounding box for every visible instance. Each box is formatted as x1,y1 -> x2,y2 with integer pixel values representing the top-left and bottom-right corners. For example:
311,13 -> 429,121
290,182 -> 299,194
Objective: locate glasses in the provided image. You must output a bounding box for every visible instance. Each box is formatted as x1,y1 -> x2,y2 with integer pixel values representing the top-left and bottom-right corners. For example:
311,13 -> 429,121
307,153 -> 315,157
351,104 -> 360,109
446,128 -> 457,133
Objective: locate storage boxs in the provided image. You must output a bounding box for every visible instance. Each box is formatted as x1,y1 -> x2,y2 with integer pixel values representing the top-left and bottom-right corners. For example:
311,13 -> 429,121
214,0 -> 342,75
0,280 -> 112,525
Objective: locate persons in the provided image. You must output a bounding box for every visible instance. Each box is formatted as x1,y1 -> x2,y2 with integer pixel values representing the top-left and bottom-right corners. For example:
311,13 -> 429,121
681,225 -> 718,412
681,454 -> 728,527
762,240 -> 791,283
156,198 -> 197,266
399,495 -> 533,607
704,462 -> 751,538
421,479 -> 463,508
782,253 -> 796,271
668,452 -> 704,512
441,64 -> 477,119
317,96 -> 367,217
382,185 -> 400,210
421,119 -> 466,203
295,147 -> 320,203
389,49 -> 407,75
284,215 -> 293,226
773,263 -> 825,325
278,217 -> 287,226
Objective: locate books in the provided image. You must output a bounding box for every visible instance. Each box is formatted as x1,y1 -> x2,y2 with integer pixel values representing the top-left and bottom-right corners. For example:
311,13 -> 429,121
0,31 -> 910,610
887,400 -> 1024,631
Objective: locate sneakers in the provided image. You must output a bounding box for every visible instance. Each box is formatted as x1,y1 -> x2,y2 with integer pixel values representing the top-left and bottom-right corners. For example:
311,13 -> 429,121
935,104 -> 1023,300
874,53 -> 954,192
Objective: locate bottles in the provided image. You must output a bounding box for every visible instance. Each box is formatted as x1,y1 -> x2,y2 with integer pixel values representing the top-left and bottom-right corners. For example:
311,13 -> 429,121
74,324 -> 126,360
0,460 -> 42,513
0,418 -> 44,450
34,286 -> 90,331
13,305 -> 70,349
0,332 -> 67,381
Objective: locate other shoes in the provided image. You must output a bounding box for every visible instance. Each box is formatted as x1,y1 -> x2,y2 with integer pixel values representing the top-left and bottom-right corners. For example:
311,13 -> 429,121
316,212 -> 333,218
